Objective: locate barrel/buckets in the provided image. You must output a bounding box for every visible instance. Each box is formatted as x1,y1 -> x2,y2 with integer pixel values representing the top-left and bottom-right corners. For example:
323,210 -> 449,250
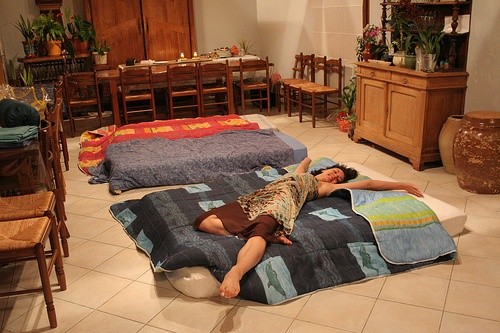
438,115 -> 464,174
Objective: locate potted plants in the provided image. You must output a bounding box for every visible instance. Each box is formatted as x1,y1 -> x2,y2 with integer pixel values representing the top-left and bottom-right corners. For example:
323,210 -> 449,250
387,0 -> 447,72
336,69 -> 357,133
2,11 -> 111,65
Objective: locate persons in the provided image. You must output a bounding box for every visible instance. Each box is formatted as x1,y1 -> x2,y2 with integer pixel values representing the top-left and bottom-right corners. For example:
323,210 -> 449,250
192,158 -> 424,300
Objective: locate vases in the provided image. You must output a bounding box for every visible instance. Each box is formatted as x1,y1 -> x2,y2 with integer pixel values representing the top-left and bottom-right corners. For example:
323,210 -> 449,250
362,53 -> 383,63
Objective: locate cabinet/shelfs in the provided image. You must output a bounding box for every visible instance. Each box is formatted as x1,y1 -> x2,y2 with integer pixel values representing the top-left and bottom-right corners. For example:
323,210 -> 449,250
353,62 -> 468,171
80,0 -> 198,70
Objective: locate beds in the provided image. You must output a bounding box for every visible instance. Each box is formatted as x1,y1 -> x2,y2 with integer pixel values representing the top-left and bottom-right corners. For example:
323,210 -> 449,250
109,156 -> 469,305
88,128 -> 307,195
78,113 -> 306,174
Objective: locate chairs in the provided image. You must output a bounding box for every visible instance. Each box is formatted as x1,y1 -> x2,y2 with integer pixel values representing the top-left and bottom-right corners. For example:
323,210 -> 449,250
0,52 -> 70,328
63,55 -> 267,138
276,51 -> 342,128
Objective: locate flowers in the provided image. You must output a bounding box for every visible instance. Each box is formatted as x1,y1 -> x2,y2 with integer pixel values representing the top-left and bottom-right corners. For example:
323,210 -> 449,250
355,24 -> 392,58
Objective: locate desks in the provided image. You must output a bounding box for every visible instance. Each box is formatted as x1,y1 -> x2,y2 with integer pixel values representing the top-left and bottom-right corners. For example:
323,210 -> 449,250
95,54 -> 274,127
